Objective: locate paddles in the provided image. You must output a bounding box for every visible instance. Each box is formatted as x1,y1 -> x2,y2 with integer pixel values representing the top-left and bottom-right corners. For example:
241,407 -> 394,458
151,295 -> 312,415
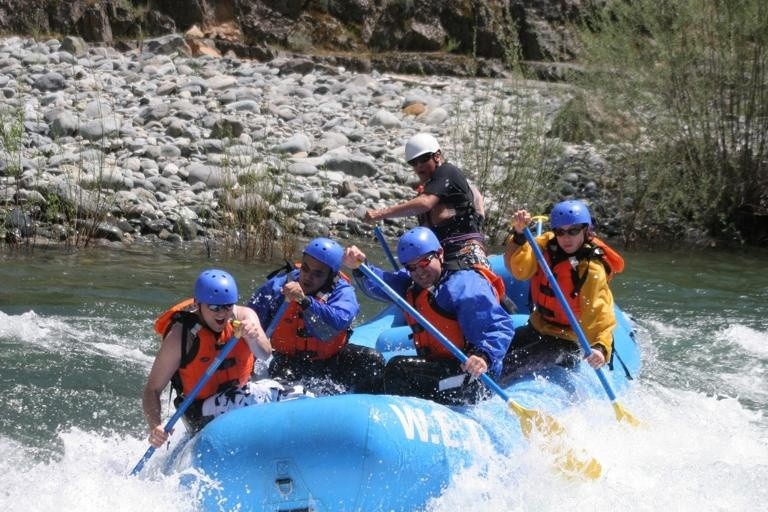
357,262 -> 602,483
522,226 -> 644,426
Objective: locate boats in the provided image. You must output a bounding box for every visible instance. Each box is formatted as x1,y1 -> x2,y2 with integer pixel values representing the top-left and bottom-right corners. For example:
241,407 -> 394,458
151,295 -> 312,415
159,252 -> 642,512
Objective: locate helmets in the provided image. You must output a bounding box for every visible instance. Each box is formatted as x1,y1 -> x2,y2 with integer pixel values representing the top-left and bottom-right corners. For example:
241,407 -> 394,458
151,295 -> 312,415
404,134 -> 441,162
396,226 -> 442,265
303,237 -> 344,275
550,200 -> 591,229
194,269 -> 237,305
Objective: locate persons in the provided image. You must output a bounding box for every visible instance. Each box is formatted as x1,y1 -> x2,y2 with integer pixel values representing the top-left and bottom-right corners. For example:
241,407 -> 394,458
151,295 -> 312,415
246,236 -> 384,395
143,268 -> 281,460
496,201 -> 616,379
344,226 -> 514,410
363,130 -> 492,274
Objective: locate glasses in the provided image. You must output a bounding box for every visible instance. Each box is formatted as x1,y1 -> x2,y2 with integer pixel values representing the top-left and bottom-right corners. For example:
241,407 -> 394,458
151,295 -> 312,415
408,155 -> 433,166
301,263 -> 323,277
207,305 -> 232,311
403,252 -> 434,271
553,224 -> 586,236
